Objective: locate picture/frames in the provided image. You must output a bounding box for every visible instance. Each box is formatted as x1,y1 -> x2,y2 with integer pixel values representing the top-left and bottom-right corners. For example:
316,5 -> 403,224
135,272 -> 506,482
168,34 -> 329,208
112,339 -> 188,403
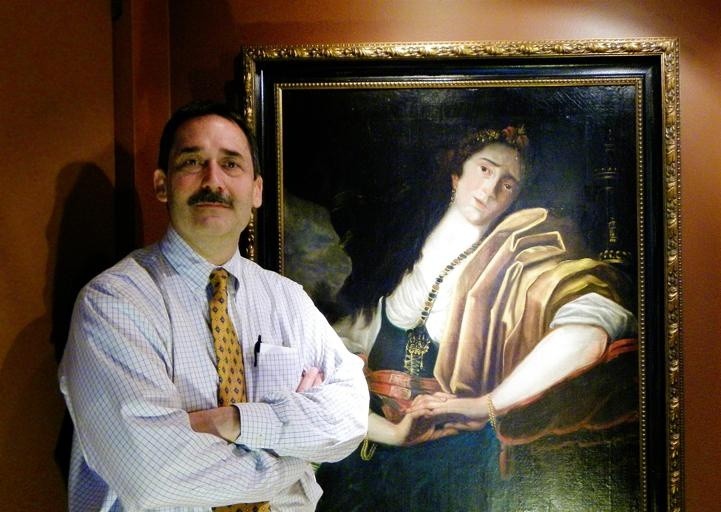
241,36 -> 686,512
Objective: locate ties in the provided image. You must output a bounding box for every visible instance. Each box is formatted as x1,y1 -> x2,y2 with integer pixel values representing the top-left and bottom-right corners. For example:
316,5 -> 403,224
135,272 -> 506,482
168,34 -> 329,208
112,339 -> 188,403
208,265 -> 273,512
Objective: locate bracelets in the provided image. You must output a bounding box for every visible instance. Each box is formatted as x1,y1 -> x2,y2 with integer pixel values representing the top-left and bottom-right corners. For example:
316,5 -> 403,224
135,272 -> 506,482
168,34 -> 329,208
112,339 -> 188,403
360,435 -> 378,461
487,392 -> 499,432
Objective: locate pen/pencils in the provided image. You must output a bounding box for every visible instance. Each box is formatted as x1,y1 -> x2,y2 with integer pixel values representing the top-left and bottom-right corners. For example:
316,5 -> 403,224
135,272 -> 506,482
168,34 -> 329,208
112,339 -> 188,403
254,335 -> 262,366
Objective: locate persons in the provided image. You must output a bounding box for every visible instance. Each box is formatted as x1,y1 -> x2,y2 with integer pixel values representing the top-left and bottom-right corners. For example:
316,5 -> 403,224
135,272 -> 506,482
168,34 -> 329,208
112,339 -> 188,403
307,121 -> 634,510
53,98 -> 369,510
